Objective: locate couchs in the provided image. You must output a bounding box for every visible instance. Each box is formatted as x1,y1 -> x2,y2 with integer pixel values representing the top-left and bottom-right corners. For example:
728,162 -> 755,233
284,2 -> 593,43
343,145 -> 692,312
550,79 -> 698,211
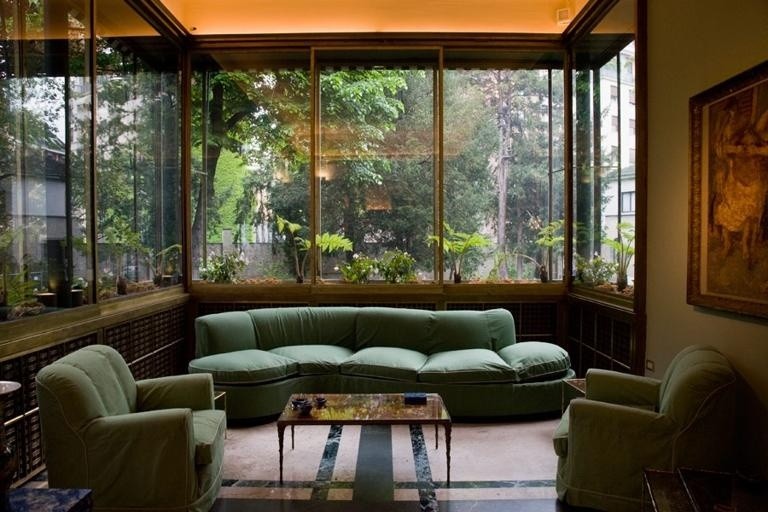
189,304 -> 577,427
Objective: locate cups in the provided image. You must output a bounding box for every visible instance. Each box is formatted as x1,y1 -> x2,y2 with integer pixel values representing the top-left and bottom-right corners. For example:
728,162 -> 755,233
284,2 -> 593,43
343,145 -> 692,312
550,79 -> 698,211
291,396 -> 327,412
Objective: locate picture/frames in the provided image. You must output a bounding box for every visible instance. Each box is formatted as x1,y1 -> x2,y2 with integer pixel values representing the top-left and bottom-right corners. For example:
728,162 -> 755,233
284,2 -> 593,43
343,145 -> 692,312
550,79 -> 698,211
689,61 -> 767,321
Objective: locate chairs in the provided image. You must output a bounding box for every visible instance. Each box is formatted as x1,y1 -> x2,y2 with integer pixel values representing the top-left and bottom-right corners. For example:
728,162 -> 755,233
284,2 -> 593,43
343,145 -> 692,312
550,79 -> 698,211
34,343 -> 229,512
554,344 -> 737,511
